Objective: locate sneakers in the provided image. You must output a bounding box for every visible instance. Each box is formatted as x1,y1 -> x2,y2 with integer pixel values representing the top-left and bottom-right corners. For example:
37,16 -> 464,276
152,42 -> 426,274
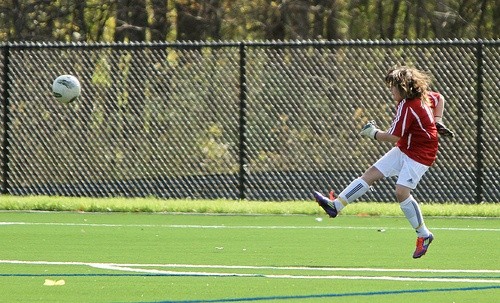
313,190 -> 338,218
413,231 -> 433,258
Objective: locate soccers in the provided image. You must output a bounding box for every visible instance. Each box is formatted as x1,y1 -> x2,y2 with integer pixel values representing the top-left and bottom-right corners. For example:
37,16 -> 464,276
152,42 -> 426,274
51,75 -> 81,104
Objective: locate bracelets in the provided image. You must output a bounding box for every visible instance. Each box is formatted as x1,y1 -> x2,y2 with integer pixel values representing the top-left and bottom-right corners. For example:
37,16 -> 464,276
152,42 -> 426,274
433,116 -> 442,123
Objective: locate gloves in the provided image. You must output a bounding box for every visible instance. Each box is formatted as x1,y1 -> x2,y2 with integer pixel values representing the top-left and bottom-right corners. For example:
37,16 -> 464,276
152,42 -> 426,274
434,116 -> 454,138
359,122 -> 381,140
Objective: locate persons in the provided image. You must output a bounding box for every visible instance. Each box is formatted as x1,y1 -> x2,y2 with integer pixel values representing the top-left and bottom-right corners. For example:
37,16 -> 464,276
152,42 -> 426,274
312,66 -> 454,259
